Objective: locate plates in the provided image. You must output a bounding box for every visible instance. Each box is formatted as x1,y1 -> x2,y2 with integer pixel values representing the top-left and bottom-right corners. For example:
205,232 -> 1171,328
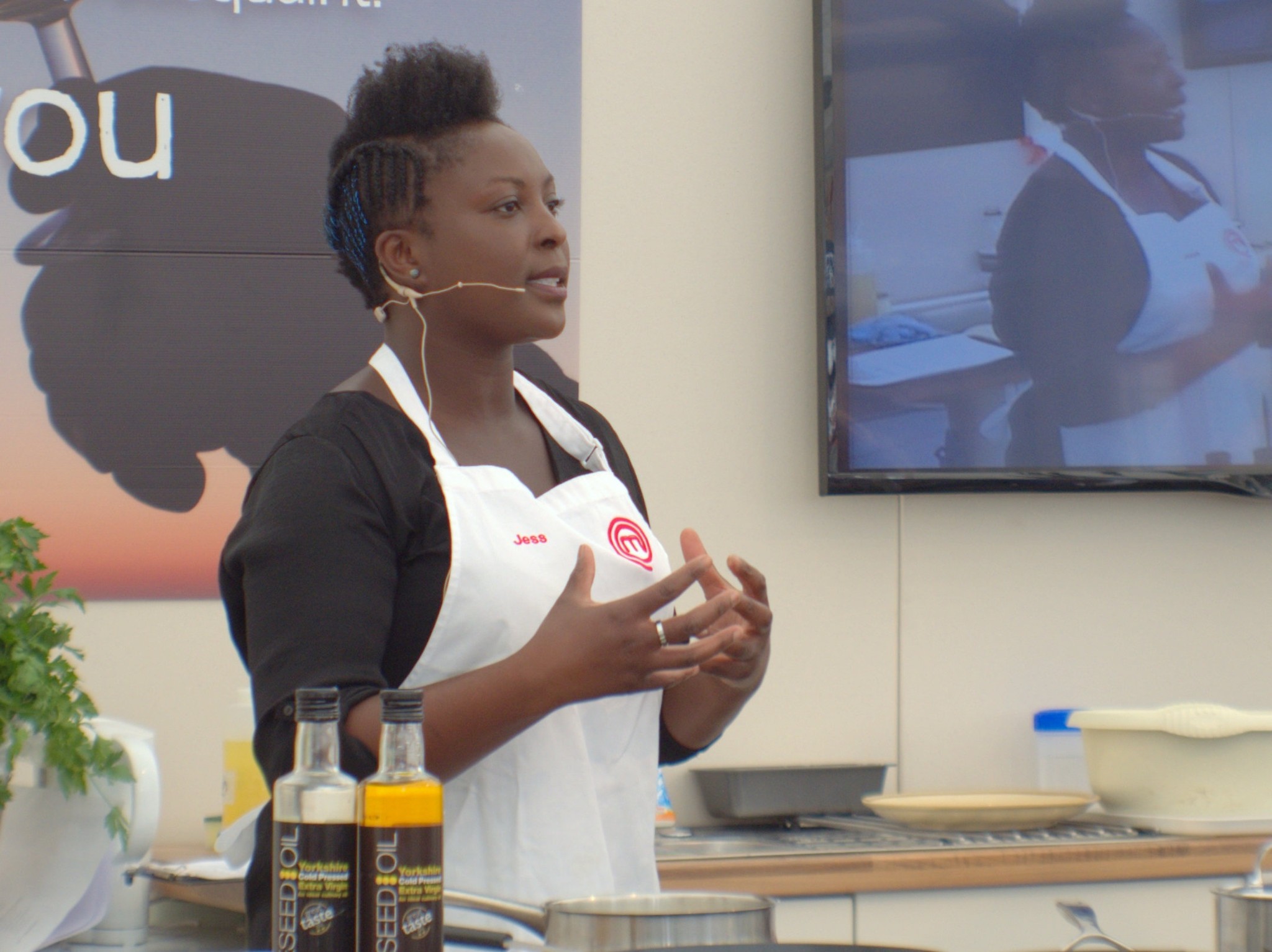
862,791 -> 1101,832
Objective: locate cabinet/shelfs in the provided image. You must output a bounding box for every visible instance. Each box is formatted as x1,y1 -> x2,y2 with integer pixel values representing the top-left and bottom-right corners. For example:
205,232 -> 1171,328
768,875 -> 1272,952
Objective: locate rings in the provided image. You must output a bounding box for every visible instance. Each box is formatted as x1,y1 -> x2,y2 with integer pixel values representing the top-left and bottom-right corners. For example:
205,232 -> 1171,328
655,622 -> 667,645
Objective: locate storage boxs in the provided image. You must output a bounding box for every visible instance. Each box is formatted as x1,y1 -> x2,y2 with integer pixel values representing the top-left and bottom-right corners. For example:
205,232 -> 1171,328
1035,703 -> 1270,835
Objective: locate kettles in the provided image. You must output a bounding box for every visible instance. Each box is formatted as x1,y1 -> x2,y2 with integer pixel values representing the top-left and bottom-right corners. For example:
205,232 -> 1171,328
32,717 -> 160,944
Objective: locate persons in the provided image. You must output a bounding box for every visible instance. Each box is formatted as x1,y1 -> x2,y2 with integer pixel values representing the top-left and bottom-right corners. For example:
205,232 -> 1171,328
218,39 -> 773,945
989,0 -> 1272,469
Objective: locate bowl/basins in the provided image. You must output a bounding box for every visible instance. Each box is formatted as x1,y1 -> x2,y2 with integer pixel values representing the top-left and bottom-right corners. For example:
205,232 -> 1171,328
1066,702 -> 1272,814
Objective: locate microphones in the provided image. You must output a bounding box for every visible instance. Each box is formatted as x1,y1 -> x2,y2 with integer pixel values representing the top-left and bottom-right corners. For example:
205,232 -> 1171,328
1092,113 -> 1175,125
402,282 -> 528,303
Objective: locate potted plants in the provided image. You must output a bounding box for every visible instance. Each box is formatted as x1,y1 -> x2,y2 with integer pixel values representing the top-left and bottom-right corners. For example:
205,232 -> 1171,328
0,513 -> 141,943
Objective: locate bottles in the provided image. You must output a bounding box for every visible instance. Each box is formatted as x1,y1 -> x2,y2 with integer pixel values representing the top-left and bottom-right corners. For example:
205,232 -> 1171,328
352,689 -> 445,952
271,688 -> 360,952
977,205 -> 1006,288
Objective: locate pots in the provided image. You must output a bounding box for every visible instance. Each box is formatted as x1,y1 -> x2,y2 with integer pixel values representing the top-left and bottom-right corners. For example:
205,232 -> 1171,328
442,892 -> 771,947
